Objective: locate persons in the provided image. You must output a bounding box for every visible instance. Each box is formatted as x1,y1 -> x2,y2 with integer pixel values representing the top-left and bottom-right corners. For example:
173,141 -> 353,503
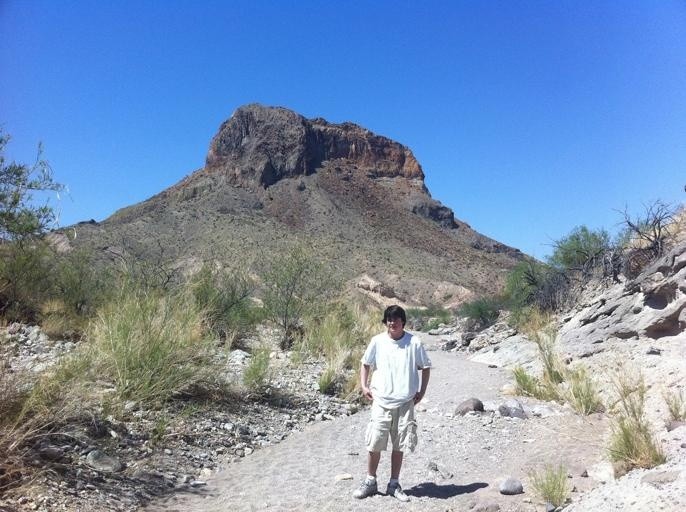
354,305 -> 431,502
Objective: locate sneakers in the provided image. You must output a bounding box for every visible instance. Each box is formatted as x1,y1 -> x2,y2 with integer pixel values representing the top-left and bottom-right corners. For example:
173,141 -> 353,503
352,480 -> 377,499
387,483 -> 410,502
